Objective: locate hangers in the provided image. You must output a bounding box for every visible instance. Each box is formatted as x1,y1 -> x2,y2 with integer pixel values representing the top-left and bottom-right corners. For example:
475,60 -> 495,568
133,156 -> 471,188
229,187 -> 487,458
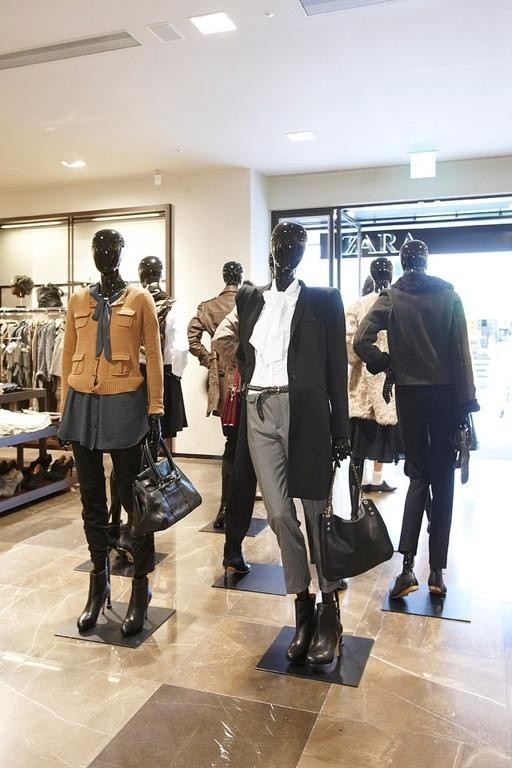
0,307 -> 62,319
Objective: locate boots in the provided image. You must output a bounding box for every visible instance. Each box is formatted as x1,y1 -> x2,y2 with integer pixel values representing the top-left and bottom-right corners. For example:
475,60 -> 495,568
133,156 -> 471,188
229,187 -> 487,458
121,576 -> 152,635
286,593 -> 344,666
77,570 -> 111,629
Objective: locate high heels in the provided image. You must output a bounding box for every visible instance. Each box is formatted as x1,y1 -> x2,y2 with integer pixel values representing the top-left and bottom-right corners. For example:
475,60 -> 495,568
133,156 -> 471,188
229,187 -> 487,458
0,454 -> 73,498
222,558 -> 251,575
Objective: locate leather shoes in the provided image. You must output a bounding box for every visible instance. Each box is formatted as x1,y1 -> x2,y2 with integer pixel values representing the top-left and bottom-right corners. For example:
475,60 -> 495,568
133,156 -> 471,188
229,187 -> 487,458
361,480 -> 397,493
390,572 -> 419,598
429,572 -> 447,592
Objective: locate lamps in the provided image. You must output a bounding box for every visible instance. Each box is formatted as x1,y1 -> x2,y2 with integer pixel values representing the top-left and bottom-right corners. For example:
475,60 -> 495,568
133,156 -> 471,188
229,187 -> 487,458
1,220 -> 63,228
154,169 -> 161,186
90,213 -> 160,222
409,149 -> 437,179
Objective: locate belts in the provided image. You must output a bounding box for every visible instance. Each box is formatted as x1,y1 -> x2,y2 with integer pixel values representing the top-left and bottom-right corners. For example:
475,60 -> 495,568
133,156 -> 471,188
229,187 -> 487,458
248,385 -> 289,423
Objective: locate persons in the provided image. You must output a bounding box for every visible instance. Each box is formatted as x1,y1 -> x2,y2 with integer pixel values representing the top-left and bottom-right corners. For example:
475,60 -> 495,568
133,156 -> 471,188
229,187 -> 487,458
58,229 -> 164,639
237,223 -> 350,669
353,239 -> 478,602
346,259 -> 403,490
212,250 -> 273,577
105,256 -> 182,565
186,262 -> 244,528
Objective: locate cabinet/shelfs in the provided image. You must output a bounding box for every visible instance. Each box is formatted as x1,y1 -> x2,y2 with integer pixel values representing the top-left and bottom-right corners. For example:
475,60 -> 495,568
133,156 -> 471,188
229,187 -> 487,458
1,387 -> 82,520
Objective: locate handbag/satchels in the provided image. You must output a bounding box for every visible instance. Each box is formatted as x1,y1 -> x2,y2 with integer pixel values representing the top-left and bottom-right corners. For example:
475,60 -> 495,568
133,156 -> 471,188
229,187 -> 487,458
221,364 -> 240,426
129,433 -> 201,538
448,412 -> 478,451
319,499 -> 394,582
